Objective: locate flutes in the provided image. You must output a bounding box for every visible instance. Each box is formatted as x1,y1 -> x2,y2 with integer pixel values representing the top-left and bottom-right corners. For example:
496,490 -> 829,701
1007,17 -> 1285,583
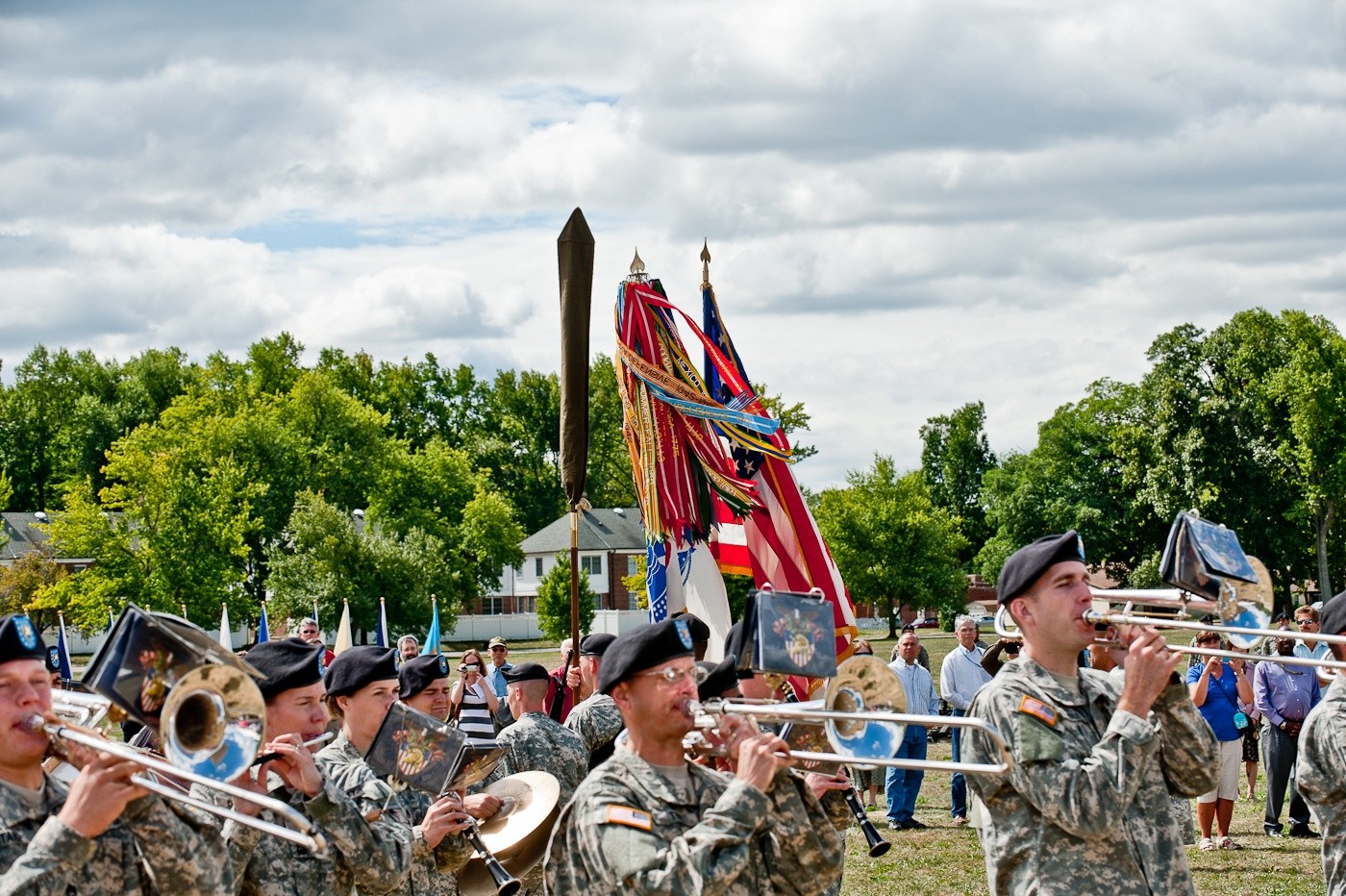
436,792 -> 521,894
780,677 -> 891,856
248,732 -> 334,767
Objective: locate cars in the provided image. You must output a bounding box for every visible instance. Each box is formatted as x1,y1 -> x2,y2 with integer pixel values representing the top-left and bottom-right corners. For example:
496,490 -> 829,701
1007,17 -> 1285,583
862,617 -> 903,631
910,618 -> 938,628
973,616 -> 995,627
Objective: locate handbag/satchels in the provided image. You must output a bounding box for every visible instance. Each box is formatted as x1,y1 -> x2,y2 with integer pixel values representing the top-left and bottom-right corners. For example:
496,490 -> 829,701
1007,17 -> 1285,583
1233,709 -> 1255,734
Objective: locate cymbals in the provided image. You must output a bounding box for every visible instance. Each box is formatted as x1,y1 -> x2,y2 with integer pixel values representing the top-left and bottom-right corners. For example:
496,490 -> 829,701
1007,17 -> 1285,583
449,770 -> 559,896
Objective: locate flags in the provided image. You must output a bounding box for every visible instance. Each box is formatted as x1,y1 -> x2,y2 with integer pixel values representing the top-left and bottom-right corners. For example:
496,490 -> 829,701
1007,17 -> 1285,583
219,608 -> 233,653
376,607 -> 388,649
420,601 -> 442,656
697,283 -> 859,679
257,610 -> 271,645
56,626 -> 73,687
617,277 -> 733,665
331,607 -> 352,658
310,610 -> 320,631
106,615 -> 114,631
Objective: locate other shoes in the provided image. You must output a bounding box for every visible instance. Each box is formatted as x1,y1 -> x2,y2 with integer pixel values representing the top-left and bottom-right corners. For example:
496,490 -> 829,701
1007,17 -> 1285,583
1199,838 -> 1216,851
1290,826 -> 1320,837
1266,829 -> 1284,837
953,815 -> 966,824
889,820 -> 902,830
865,803 -> 878,811
1216,836 -> 1241,850
902,818 -> 926,829
1237,793 -> 1243,801
1247,793 -> 1255,802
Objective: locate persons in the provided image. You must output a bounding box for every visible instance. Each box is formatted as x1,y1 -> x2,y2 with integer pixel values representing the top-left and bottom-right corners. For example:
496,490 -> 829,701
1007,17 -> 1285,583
39,616 -> 848,886
542,618 -> 844,895
1293,590 -> 1346,896
315,645 -> 483,895
397,655 -> 503,821
465,663 -> 592,896
850,614 -> 1133,831
188,637 -> 417,895
0,614 -> 243,896
1180,600 -> 1331,853
563,634 -> 624,764
959,527 -> 1221,896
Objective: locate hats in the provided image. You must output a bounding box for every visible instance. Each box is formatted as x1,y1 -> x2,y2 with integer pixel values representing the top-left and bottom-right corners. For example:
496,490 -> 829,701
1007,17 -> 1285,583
489,637 -> 507,648
1321,590 -> 1346,634
0,613 -> 61,671
1200,614 -> 1212,622
996,530 -> 1085,605
902,623 -> 914,634
239,612 -> 738,703
1275,613 -> 1289,622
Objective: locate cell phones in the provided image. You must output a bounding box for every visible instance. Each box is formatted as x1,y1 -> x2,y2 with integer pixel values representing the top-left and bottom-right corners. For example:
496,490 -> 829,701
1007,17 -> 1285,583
1222,656 -> 1231,662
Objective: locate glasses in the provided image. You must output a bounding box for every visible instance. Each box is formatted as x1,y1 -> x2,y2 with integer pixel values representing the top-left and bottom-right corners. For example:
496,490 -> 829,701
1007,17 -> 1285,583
303,630 -> 316,634
1200,635 -> 1219,642
1297,618 -> 1316,625
631,665 -> 708,683
492,648 -> 504,652
463,662 -> 479,669
854,652 -> 872,655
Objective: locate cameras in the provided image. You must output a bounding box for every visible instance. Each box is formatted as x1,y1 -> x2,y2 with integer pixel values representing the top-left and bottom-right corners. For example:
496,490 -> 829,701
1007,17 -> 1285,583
1004,642 -> 1022,654
462,665 -> 477,673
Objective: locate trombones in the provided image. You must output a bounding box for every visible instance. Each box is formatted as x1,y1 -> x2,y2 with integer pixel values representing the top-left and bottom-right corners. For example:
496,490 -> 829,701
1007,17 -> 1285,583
19,663 -> 326,856
996,554 -> 1346,670
675,656 -> 1013,778
